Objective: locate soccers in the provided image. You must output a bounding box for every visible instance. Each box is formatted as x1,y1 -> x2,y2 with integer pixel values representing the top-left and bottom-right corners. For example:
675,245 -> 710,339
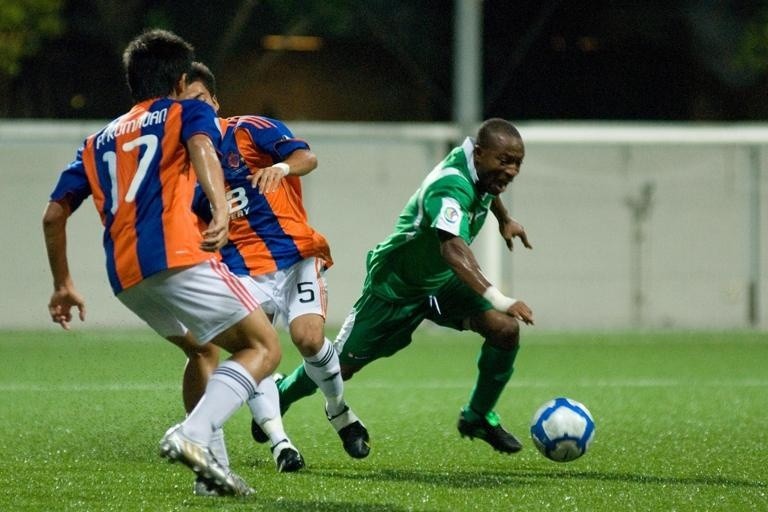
531,398 -> 595,462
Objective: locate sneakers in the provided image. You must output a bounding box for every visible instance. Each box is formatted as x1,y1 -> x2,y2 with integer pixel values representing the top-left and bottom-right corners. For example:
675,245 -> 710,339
251,371 -> 287,444
270,439 -> 305,473
324,401 -> 372,459
156,424 -> 258,497
454,404 -> 524,455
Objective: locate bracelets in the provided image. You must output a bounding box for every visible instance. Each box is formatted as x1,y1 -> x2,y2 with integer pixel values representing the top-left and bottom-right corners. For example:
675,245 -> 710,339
273,162 -> 290,176
483,286 -> 517,313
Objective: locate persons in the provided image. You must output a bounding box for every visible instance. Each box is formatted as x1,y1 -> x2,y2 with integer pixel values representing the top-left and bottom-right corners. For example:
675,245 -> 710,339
44,30 -> 281,496
180,62 -> 371,472
252,118 -> 534,453
